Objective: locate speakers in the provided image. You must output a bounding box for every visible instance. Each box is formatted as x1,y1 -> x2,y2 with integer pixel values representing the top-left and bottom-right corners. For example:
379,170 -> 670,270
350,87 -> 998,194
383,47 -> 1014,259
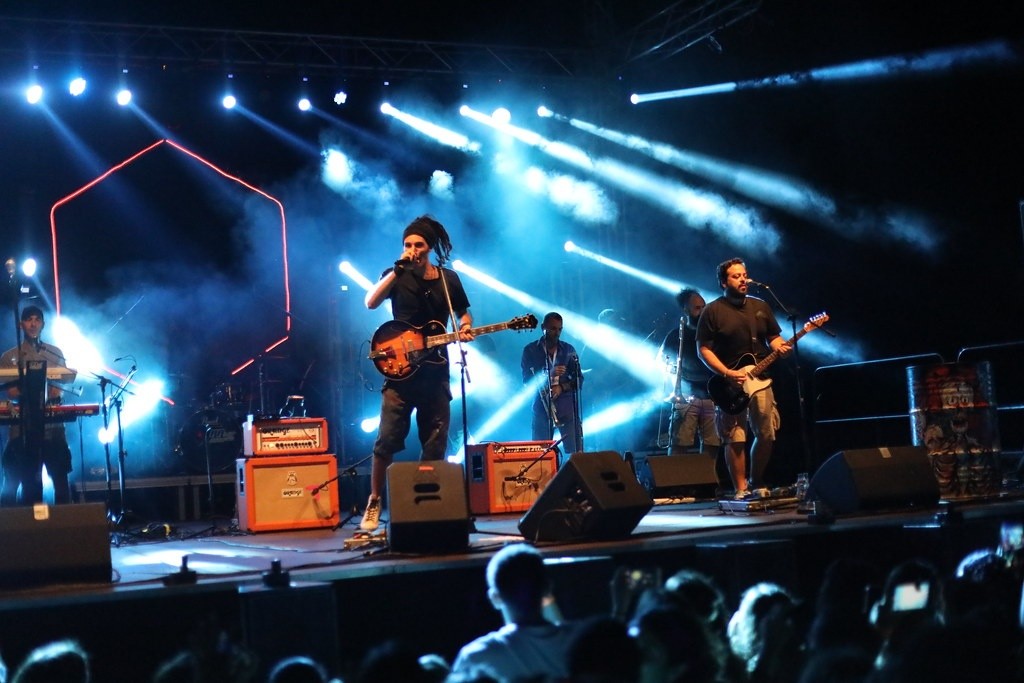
518,449 -> 720,543
384,460 -> 469,552
806,446 -> 940,516
235,453 -> 340,533
467,440 -> 558,516
0,502 -> 112,590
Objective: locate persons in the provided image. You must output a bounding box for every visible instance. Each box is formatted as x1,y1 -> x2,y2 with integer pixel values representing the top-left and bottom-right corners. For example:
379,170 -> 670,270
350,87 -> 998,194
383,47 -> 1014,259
695,258 -> 793,501
520,312 -> 583,454
662,288 -> 720,455
0,542 -> 1024,683
359,216 -> 474,533
0,306 -> 73,508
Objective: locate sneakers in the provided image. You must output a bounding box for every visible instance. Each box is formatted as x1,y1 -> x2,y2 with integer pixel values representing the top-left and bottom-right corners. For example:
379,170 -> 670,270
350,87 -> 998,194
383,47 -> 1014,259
360,495 -> 382,531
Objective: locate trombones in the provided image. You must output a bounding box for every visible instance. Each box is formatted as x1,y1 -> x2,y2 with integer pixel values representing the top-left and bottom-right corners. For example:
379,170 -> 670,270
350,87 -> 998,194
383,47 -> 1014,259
657,315 -> 689,450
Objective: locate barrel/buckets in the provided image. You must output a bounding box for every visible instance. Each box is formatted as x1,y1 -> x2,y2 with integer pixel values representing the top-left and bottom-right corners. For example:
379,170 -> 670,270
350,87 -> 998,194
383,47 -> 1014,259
905,359 -> 1002,499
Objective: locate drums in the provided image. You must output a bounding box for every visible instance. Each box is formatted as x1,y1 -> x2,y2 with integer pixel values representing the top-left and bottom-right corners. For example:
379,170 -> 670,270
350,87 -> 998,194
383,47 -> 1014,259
173,400 -> 243,471
214,381 -> 249,410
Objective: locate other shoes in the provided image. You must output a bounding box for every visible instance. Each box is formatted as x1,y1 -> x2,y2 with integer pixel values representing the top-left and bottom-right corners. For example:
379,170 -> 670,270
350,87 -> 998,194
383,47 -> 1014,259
735,490 -> 752,500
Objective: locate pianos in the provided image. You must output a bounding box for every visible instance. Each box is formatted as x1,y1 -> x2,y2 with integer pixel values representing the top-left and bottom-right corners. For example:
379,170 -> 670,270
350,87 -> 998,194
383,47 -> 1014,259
1,405 -> 100,510
0,367 -> 78,382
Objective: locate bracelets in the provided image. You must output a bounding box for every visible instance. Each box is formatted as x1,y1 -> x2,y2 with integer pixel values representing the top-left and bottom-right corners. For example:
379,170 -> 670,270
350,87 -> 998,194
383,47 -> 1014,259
724,369 -> 731,379
459,322 -> 472,331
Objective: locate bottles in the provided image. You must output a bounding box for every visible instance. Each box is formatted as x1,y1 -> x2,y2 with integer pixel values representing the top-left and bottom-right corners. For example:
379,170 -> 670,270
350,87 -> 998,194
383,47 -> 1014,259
796,473 -> 814,512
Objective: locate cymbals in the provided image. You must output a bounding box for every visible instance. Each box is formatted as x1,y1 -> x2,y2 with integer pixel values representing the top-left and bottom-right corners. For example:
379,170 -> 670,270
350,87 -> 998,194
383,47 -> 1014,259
237,350 -> 291,360
164,371 -> 193,380
229,376 -> 282,384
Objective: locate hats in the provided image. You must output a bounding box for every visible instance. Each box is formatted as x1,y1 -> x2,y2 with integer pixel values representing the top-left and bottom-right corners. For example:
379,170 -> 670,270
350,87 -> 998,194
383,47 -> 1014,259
21,305 -> 44,322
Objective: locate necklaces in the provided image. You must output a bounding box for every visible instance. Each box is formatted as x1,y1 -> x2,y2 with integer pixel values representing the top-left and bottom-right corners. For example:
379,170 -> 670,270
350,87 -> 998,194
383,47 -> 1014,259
413,264 -> 435,299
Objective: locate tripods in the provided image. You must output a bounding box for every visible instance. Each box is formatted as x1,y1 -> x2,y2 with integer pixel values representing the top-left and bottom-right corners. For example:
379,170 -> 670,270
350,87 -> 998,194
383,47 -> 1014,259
317,453 -> 387,531
181,426 -> 225,541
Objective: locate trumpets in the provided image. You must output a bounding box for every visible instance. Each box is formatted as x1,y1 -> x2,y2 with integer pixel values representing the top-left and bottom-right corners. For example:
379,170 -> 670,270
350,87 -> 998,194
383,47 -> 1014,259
529,367 -> 565,429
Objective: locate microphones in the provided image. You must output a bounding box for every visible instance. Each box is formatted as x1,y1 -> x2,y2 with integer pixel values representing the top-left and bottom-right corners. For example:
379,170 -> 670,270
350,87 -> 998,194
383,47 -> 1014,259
5,259 -> 16,276
570,352 -> 577,362
746,279 -> 768,289
31,334 -> 40,353
311,489 -> 319,495
504,476 -> 527,481
394,258 -> 410,265
543,329 -> 549,339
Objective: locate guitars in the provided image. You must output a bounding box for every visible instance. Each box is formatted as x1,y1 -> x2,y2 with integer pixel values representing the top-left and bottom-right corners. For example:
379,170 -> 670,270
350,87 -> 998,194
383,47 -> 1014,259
708,312 -> 829,415
371,314 -> 539,382
